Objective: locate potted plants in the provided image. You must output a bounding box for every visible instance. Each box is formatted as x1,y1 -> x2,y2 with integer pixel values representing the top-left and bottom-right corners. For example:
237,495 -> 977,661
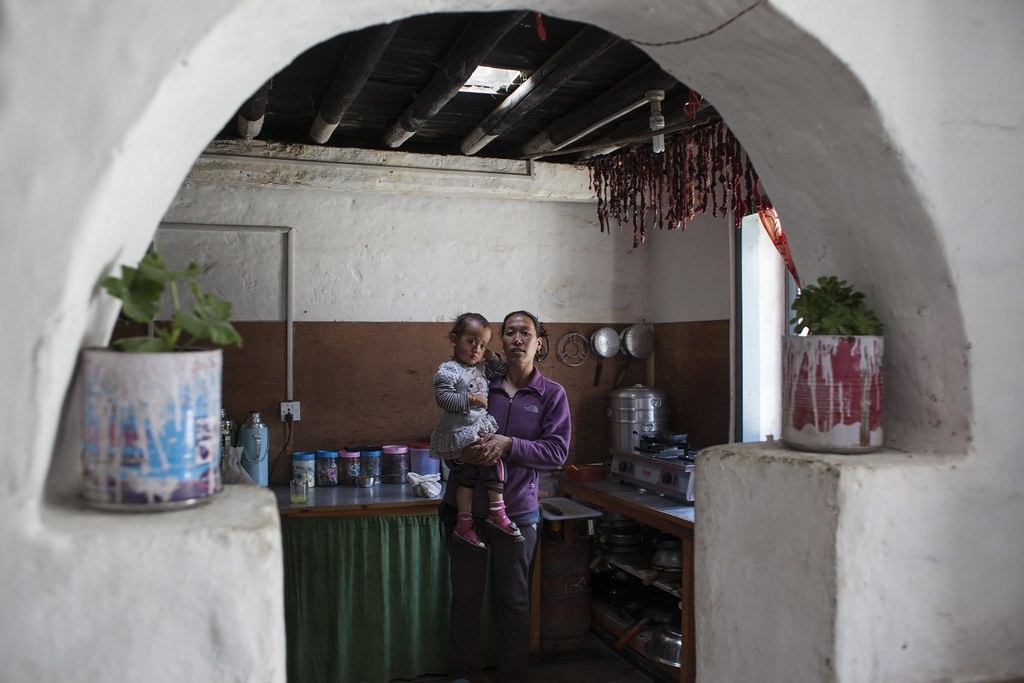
781,276 -> 887,453
69,240 -> 244,517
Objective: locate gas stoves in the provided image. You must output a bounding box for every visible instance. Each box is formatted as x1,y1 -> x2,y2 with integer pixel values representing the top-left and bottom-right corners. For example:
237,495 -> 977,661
609,447 -> 700,506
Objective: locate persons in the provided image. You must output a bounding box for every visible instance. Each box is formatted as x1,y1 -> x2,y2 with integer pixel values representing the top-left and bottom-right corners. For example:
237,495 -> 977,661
429,313 -> 526,550
437,311 -> 572,683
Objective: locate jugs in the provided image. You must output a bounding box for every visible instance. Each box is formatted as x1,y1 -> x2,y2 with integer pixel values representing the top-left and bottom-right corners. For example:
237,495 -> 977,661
239,411 -> 269,487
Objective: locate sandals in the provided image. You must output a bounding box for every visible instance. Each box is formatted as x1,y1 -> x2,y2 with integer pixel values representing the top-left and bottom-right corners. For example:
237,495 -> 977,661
485,513 -> 525,542
453,522 -> 487,554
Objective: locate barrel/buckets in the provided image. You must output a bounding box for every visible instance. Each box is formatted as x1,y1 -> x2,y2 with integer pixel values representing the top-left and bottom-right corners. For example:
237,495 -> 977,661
606,384 -> 671,456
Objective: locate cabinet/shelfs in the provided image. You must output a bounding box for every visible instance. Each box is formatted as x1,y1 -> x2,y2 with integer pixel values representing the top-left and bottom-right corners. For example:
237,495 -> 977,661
555,469 -> 697,682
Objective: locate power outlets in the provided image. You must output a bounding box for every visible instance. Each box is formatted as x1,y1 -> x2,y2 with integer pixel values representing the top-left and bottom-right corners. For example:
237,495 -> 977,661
281,401 -> 301,422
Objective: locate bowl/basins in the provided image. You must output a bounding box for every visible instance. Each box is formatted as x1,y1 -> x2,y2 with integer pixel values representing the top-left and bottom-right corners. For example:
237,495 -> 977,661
648,623 -> 683,668
358,476 -> 375,488
651,541 -> 683,572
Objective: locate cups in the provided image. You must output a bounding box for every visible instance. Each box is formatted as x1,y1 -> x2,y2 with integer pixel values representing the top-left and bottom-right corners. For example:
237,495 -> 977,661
290,479 -> 307,505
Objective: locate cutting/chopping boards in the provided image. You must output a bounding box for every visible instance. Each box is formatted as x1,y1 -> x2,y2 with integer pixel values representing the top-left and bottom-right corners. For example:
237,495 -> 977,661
539,496 -> 603,520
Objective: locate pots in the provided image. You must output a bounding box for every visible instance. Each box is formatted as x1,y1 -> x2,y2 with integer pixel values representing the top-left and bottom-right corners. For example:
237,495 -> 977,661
633,430 -> 688,442
590,328 -> 620,386
615,324 -> 656,387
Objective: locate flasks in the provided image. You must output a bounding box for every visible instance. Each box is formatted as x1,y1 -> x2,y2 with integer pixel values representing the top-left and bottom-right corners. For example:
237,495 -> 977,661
219,408 -> 231,473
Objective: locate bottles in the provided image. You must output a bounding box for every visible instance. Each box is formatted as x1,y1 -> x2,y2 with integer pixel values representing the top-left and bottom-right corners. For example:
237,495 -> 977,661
292,443 -> 450,488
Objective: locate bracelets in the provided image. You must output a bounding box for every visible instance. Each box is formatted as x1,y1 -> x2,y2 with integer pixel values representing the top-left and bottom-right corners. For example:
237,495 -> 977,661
470,393 -> 475,404
487,352 -> 494,362
454,459 -> 462,465
